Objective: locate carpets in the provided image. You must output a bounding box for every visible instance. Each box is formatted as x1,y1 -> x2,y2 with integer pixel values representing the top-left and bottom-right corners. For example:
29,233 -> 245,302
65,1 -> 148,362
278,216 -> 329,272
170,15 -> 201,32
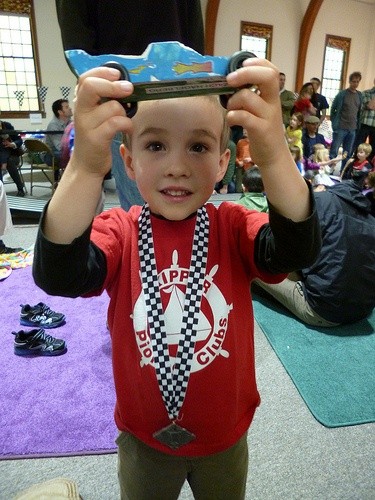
0,263 -> 118,458
250,282 -> 375,428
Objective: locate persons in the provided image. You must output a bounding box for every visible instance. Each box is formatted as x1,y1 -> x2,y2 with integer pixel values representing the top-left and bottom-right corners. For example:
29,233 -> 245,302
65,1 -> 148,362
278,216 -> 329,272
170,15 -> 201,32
0,120 -> 26,198
254,175 -> 375,327
44,99 -> 73,167
213,72 -> 375,213
31,57 -> 320,500
0,181 -> 24,254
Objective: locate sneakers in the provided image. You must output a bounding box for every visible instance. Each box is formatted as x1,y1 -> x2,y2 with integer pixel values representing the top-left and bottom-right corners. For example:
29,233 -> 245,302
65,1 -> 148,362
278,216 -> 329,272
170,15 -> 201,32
19,302 -> 66,328
12,329 -> 67,357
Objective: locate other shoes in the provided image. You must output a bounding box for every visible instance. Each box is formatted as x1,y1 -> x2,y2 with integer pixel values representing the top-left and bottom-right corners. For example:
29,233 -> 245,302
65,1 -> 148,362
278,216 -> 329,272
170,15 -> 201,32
18,187 -> 25,196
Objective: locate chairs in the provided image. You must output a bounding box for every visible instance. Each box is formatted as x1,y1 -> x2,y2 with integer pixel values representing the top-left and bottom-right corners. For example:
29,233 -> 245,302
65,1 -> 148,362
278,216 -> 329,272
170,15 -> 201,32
26,141 -> 59,196
2,145 -> 26,193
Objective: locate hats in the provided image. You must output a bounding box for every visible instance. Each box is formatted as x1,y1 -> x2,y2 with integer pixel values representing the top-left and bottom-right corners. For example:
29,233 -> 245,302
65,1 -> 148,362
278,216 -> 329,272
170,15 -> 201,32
306,115 -> 321,123
